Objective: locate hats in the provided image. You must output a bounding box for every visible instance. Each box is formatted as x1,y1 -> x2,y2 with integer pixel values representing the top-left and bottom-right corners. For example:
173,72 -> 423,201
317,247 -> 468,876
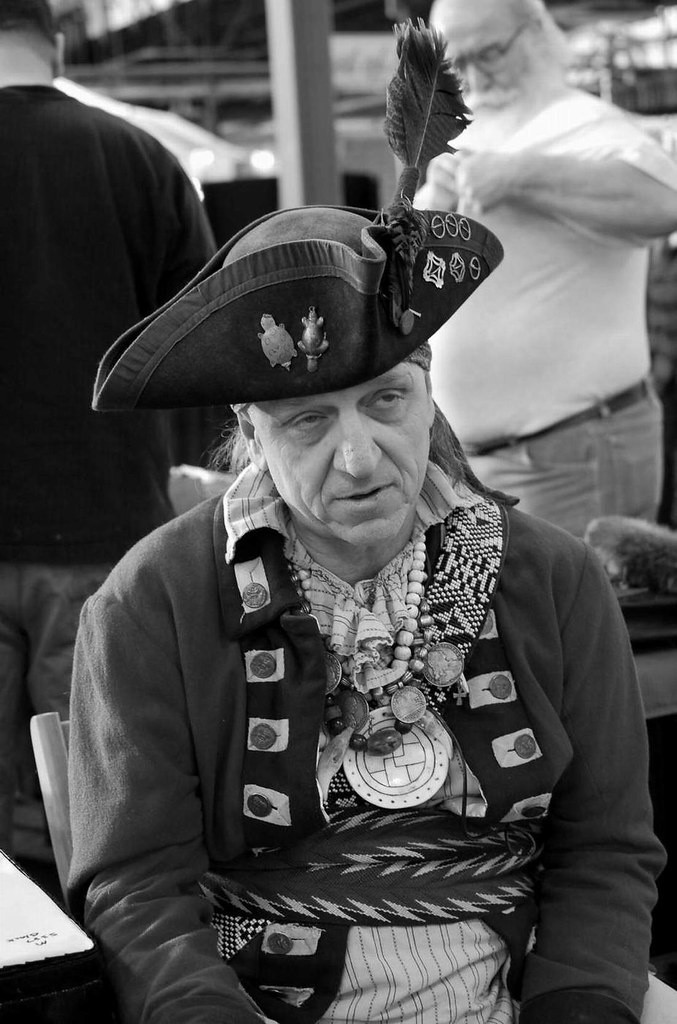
92,16 -> 503,413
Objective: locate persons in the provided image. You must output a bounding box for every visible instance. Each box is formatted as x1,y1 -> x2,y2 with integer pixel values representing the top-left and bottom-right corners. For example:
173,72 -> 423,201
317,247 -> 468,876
0,0 -> 219,900
413,0 -> 677,539
66,205 -> 666,1024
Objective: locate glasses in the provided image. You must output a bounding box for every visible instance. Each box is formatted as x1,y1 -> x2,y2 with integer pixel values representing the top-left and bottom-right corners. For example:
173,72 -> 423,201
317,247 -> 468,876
447,19 -> 534,90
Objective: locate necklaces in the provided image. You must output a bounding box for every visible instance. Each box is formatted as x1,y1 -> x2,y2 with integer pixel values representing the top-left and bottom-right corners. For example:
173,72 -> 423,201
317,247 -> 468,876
284,533 -> 464,756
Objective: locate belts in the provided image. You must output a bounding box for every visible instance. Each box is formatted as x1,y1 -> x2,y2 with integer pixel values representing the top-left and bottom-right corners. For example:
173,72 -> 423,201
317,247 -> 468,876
459,374 -> 654,456
